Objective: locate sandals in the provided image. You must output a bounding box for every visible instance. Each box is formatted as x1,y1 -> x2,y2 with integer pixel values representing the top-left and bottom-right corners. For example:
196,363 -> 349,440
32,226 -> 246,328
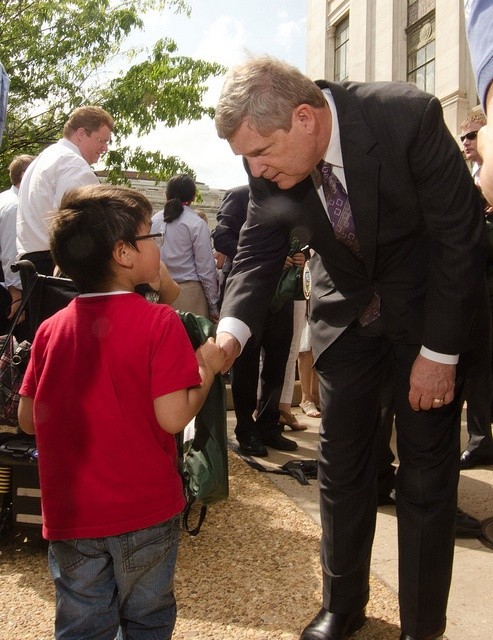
279,410 -> 308,433
300,400 -> 323,419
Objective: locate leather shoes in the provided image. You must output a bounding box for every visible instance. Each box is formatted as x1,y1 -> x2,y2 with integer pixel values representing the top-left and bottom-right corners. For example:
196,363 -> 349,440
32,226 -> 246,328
400,617 -> 449,640
267,433 -> 299,452
299,605 -> 368,640
238,433 -> 270,459
457,448 -> 492,470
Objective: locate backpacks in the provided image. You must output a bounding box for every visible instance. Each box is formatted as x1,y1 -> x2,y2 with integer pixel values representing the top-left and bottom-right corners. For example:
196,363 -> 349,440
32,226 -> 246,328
170,310 -> 230,535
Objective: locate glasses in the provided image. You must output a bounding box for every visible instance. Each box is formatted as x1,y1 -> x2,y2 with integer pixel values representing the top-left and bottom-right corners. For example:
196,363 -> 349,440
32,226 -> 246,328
83,128 -> 111,147
125,229 -> 165,249
460,130 -> 480,143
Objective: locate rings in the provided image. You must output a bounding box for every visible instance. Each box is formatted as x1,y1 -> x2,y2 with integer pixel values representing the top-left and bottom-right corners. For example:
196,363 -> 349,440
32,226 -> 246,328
432,399 -> 442,404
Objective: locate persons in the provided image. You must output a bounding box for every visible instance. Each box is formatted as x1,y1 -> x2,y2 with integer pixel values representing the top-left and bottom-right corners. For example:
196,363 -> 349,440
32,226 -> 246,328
216,56 -> 484,637
0,154 -> 37,321
150,174 -> 219,323
18,185 -> 226,640
460,106 -> 493,187
15,107 -> 112,332
213,187 -> 304,454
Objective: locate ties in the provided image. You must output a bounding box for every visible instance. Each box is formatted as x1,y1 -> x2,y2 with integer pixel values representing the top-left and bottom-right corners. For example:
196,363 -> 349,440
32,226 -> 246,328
316,158 -> 367,263
